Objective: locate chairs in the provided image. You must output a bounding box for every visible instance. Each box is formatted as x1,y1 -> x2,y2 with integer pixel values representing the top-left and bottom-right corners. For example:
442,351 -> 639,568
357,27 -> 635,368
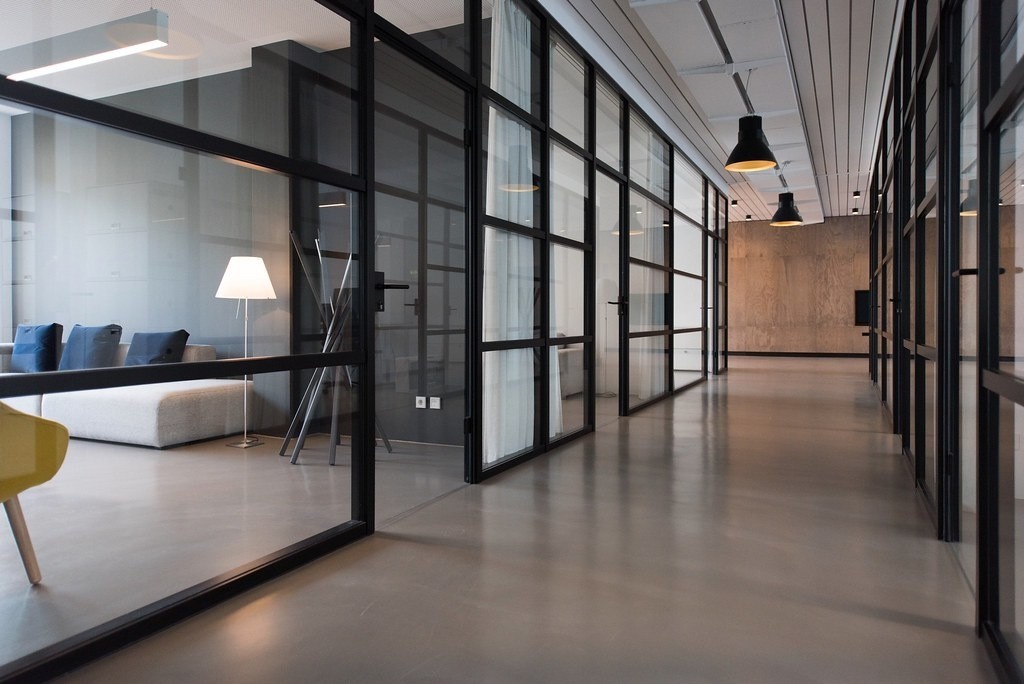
0,402 -> 70,584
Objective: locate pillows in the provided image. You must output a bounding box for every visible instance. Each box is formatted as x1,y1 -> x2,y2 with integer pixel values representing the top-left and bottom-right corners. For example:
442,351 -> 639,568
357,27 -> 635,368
557,332 -> 567,349
8,322 -> 63,373
124,329 -> 191,366
58,324 -> 123,370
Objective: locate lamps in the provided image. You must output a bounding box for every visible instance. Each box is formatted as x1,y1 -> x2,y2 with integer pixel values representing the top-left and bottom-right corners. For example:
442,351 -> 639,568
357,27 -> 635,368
215,256 -> 277,450
769,180 -> 804,227
853,191 -> 860,199
377,238 -> 392,247
852,208 -> 858,214
610,204 -> 644,236
731,200 -> 738,207
662,221 -> 669,227
596,279 -> 619,398
746,215 -> 751,221
0,0 -> 169,81
724,70 -> 778,172
318,191 -> 346,208
496,145 -> 540,192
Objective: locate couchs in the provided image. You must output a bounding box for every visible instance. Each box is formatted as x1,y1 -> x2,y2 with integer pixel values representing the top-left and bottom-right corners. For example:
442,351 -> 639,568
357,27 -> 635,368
557,344 -> 583,401
0,342 -> 254,450
